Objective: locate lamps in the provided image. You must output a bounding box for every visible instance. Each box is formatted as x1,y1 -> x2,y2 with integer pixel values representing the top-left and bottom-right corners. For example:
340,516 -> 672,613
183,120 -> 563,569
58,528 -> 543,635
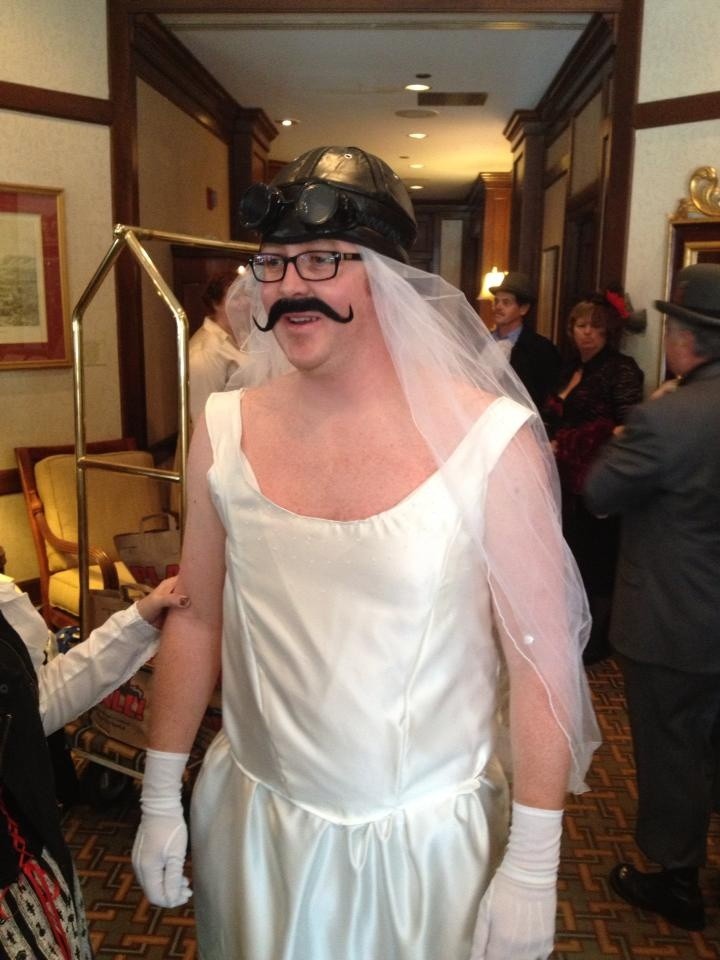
476,266 -> 510,333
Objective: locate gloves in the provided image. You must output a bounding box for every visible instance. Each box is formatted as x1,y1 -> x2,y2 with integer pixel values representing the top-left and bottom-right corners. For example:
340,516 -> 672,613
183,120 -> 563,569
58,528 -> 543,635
468,809 -> 566,960
131,746 -> 193,909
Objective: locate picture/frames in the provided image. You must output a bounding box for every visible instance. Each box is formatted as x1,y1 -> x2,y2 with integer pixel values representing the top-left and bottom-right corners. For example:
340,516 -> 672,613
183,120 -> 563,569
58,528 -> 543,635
0,181 -> 75,370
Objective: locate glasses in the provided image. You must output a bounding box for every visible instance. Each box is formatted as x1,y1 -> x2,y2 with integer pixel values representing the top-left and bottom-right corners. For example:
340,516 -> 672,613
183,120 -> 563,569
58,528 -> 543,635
249,253 -> 364,284
240,180 -> 339,230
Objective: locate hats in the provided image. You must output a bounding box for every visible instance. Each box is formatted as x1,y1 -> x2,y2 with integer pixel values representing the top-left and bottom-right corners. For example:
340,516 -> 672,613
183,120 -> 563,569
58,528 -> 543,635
489,272 -> 537,304
655,263 -> 720,328
260,145 -> 417,267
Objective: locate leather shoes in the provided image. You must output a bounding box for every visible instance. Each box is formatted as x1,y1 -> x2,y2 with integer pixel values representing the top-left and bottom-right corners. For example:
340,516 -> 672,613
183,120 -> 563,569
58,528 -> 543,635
609,864 -> 703,929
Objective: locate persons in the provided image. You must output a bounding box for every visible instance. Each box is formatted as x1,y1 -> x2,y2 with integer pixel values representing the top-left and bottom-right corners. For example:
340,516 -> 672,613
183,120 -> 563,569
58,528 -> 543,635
579,262 -> 720,930
131,145 -> 601,959
171,272 -> 251,512
525,292 -> 645,663
467,272 -> 555,415
0,544 -> 48,673
0,576 -> 190,960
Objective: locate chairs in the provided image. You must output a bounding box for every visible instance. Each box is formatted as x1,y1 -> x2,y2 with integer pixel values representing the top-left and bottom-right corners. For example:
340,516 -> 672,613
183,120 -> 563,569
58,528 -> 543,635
12,435 -> 181,632
